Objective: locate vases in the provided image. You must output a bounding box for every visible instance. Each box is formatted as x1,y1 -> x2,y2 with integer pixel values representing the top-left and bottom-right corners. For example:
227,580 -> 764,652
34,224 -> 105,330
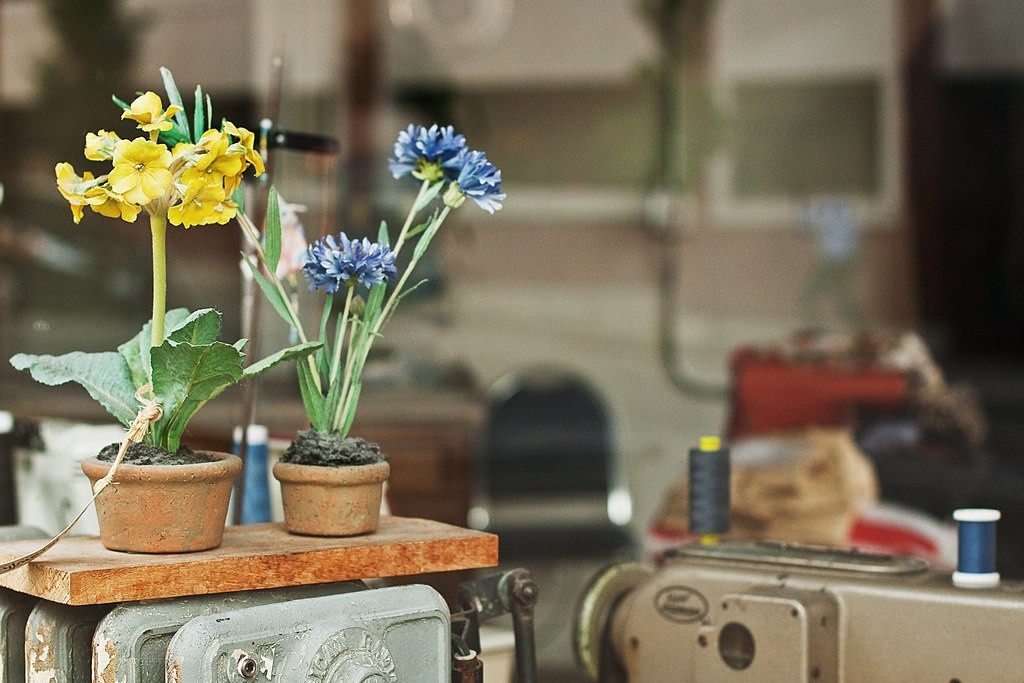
267,451 -> 393,539
78,442 -> 245,556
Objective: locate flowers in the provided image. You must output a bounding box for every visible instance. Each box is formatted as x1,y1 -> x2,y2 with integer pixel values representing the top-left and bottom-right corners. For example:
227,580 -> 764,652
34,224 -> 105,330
221,122 -> 507,443
8,88 -> 325,458
